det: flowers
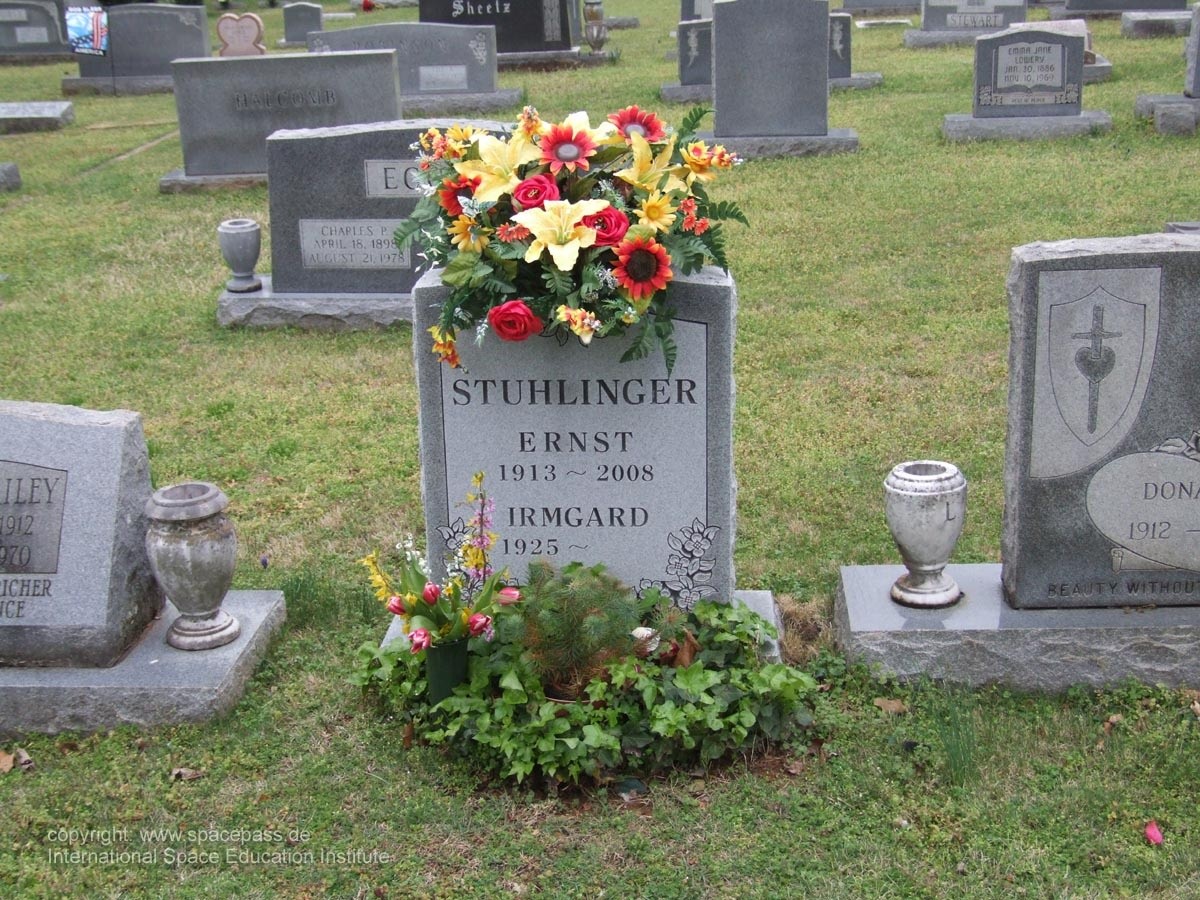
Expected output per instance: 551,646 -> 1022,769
390,104 -> 750,379
356,470 -> 521,654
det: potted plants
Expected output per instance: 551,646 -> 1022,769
498,560 -> 688,718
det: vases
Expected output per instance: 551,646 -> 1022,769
424,631 -> 472,743
